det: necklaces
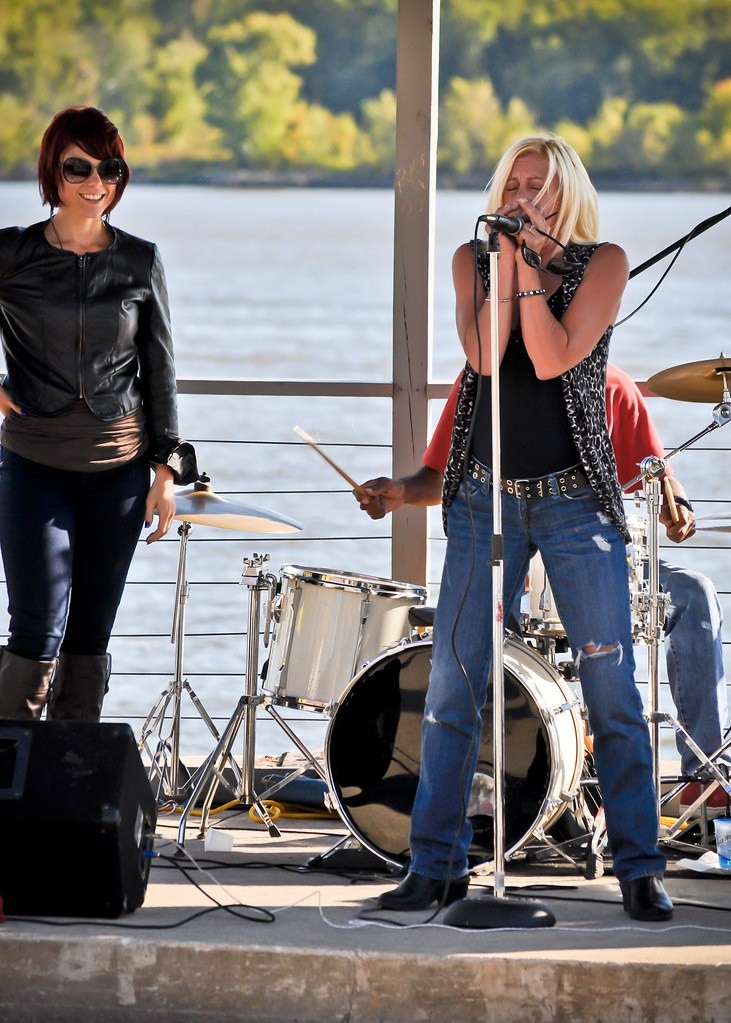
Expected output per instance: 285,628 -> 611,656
50,215 -> 65,250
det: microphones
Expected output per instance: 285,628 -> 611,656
479,214 -> 526,237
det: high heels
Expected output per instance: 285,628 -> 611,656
378,872 -> 471,911
621,877 -> 675,921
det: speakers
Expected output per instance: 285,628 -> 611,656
0,718 -> 159,919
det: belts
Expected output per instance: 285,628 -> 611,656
466,456 -> 589,501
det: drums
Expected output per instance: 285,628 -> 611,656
263,562 -> 429,714
323,633 -> 584,875
529,514 -> 647,632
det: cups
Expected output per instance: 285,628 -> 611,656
713,817 -> 731,870
203,827 -> 233,852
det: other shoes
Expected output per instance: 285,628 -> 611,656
678,781 -> 730,815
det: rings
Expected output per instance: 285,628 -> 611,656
528,225 -> 535,233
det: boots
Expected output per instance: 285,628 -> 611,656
46,650 -> 111,721
0,645 -> 59,719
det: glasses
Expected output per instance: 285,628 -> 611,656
521,212 -> 584,277
57,157 -> 125,185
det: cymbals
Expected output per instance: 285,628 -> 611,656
645,358 -> 731,403
152,490 -> 305,533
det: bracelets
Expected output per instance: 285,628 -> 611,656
515,286 -> 545,297
483,298 -> 511,303
666,495 -> 693,512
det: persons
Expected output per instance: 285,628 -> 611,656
353,362 -> 731,824
378,135 -> 678,920
0,107 -> 199,720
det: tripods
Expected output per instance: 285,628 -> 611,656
584,370 -> 731,880
135,520 -> 332,856
